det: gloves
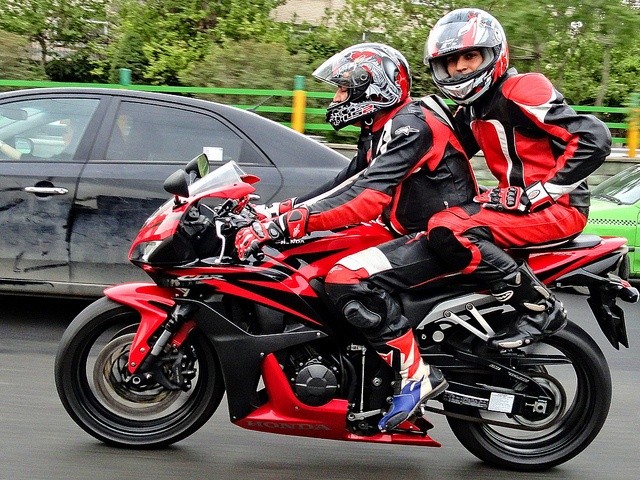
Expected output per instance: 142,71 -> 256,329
234,211 -> 291,261
248,201 -> 280,219
473,186 -> 530,214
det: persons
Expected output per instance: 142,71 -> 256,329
234,42 -> 479,433
425,7 -> 612,353
0,118 -> 86,161
114,115 -> 166,163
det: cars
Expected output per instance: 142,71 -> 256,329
0,85 -> 354,305
579,164 -> 640,277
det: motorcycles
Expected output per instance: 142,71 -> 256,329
53,153 -> 639,470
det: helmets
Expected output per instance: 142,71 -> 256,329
310,42 -> 413,133
422,7 -> 510,107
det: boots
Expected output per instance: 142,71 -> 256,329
369,328 -> 449,433
485,256 -> 569,350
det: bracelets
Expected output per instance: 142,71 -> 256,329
0,140 -> 5,149
